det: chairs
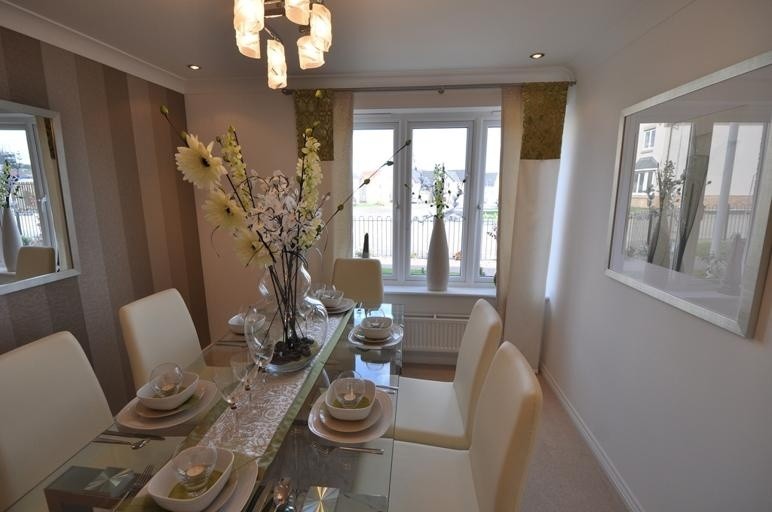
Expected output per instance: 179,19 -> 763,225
365,299 -> 502,449
118,288 -> 244,424
3,293 -> 405,512
0,330 -> 188,511
331,259 -> 383,309
16,246 -> 56,280
326,341 -> 542,511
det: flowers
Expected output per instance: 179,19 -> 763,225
403,163 -> 468,220
0,160 -> 24,207
158,103 -> 413,354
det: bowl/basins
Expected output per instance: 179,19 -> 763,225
334,369 -> 366,408
227,312 -> 266,335
150,363 -> 183,397
325,378 -> 377,421
172,436 -> 217,497
360,317 -> 393,339
316,290 -> 344,307
365,308 -> 385,327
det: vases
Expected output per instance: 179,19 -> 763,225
1,205 -> 24,271
244,252 -> 325,375
427,216 -> 450,292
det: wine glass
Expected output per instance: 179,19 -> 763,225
229,350 -> 261,415
249,336 -> 275,395
213,370 -> 247,435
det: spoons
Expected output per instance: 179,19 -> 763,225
272,485 -> 289,512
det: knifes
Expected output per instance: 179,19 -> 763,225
102,430 -> 166,440
250,478 -> 274,512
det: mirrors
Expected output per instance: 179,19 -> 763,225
604,53 -> 772,340
0,100 -> 82,297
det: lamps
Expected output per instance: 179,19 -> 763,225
232,0 -> 334,90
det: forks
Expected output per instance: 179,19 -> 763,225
112,464 -> 153,511
311,441 -> 384,456
93,436 -> 149,450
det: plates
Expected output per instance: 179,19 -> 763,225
307,388 -> 394,443
202,466 -> 241,511
130,451 -> 259,512
319,397 -> 383,432
136,372 -> 200,411
147,446 -> 234,512
135,383 -> 206,418
328,298 -> 353,314
347,325 -> 403,349
354,326 -> 391,343
116,379 -> 217,431
326,299 -> 349,311
